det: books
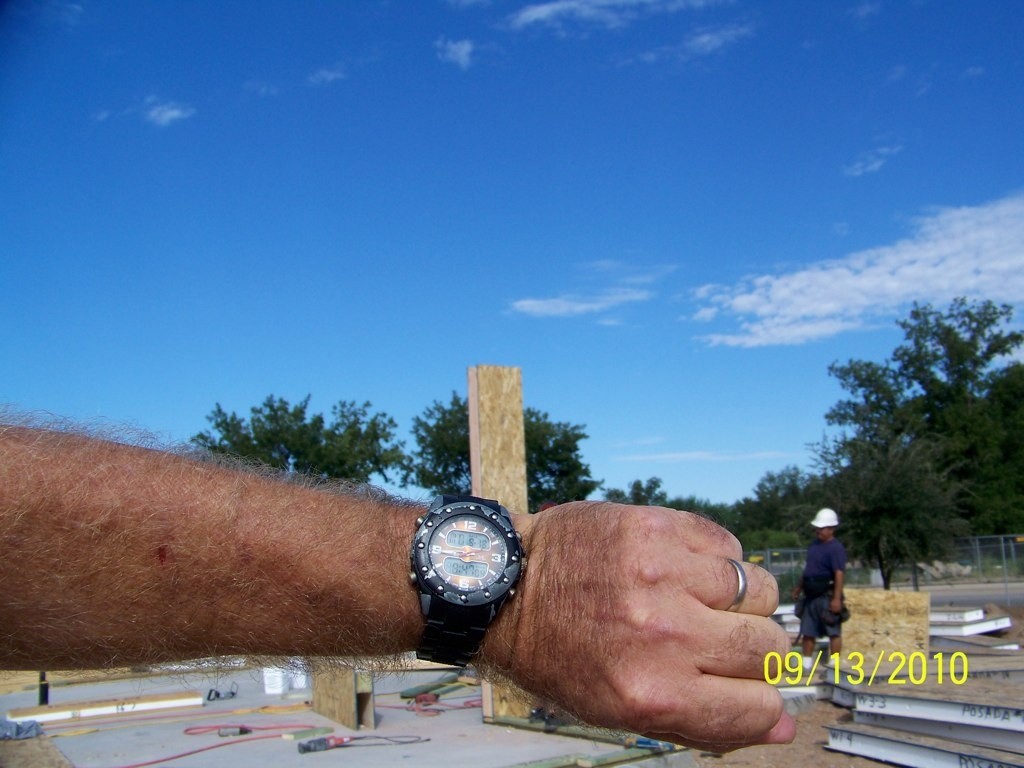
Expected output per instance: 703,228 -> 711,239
770,599 -> 1024,767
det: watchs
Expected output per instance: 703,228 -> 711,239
407,489 -> 530,672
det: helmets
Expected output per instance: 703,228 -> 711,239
810,507 -> 839,528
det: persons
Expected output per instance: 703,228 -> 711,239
791,506 -> 852,671
1,419 -> 800,757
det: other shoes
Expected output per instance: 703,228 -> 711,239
824,656 -> 838,667
801,657 -> 814,670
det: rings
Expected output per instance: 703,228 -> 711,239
724,557 -> 748,612
